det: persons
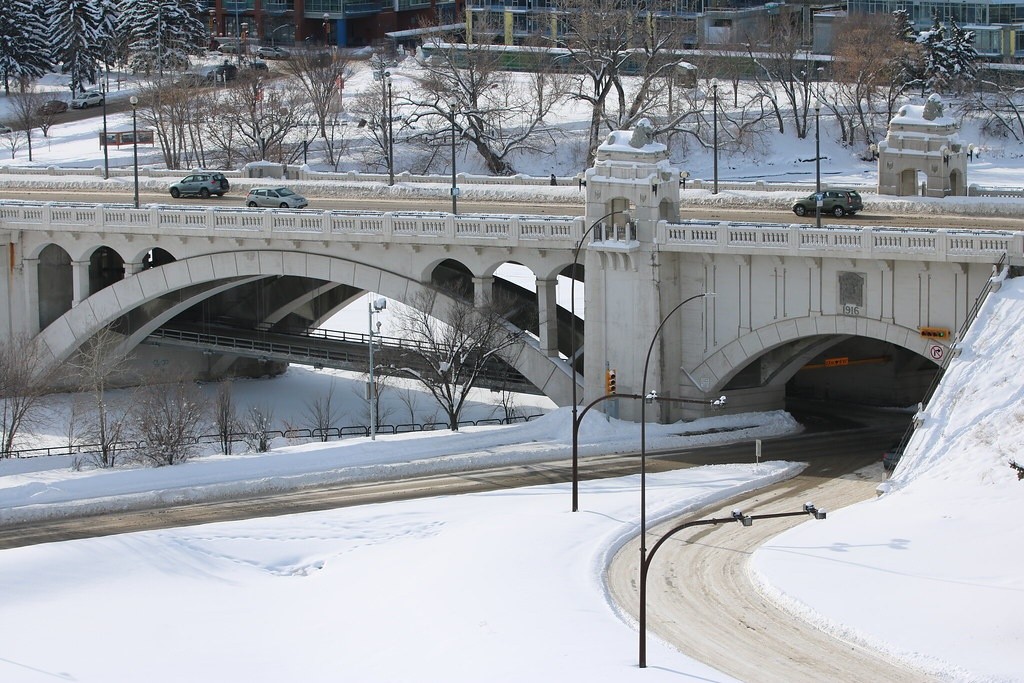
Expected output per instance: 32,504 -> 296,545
551,174 -> 557,186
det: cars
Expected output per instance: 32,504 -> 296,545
37,100 -> 67,114
217,42 -> 245,53
257,47 -> 290,60
0,124 -> 13,133
208,65 -> 238,81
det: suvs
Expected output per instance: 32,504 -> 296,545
72,92 -> 105,109
241,63 -> 268,80
792,188 -> 864,217
169,172 -> 229,199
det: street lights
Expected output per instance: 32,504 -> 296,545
711,77 -> 719,194
448,97 -> 459,216
302,136 -> 307,164
640,291 -> 719,668
815,101 -> 821,229
272,24 -> 289,47
323,13 -> 330,51
570,210 -> 631,512
129,96 -> 140,209
386,76 -> 395,186
260,131 -> 267,160
100,77 -> 109,179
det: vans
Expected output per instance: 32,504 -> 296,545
245,188 -> 308,209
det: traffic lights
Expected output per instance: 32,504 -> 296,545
604,368 -> 617,396
920,326 -> 950,339
